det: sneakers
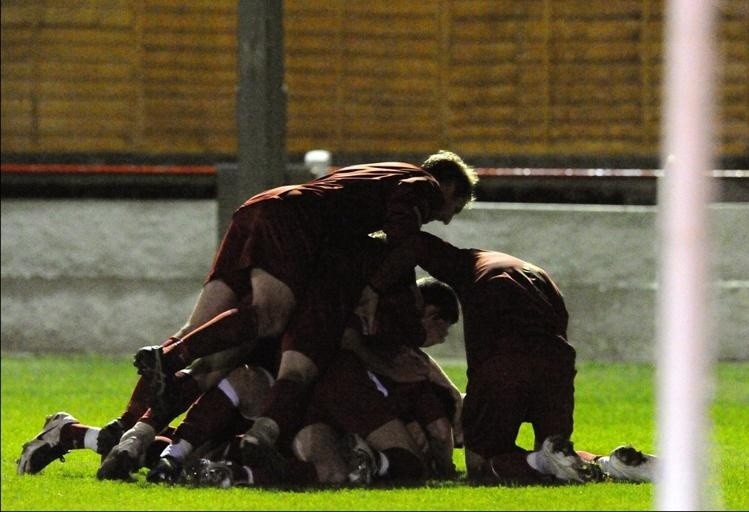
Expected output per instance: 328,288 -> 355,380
18,413 -> 80,473
541,437 -> 655,482
133,345 -> 176,413
96,420 -> 260,486
344,430 -> 381,486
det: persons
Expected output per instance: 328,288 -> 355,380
410,231 -> 659,489
16,149 -> 478,492
